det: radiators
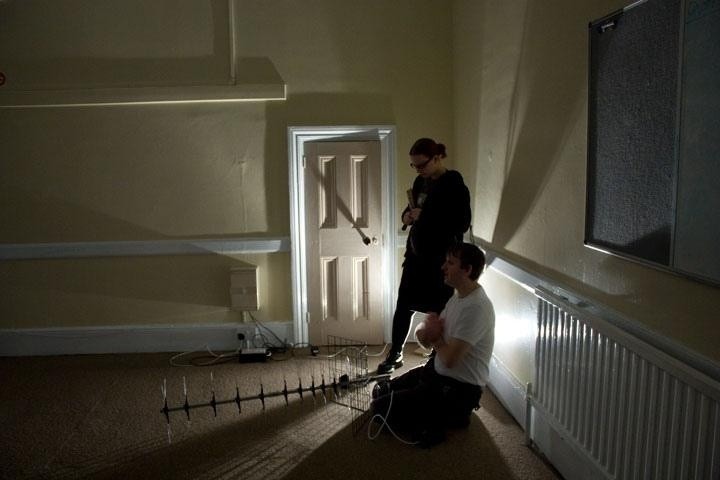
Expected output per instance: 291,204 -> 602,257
524,285 -> 720,480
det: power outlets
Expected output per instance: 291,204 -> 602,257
236,326 -> 256,340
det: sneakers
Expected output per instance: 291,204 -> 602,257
378,350 -> 403,373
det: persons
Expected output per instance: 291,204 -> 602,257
377,138 -> 471,374
375,243 -> 495,430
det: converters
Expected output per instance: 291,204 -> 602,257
239,348 -> 268,363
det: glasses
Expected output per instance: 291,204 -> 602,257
410,157 -> 432,169
